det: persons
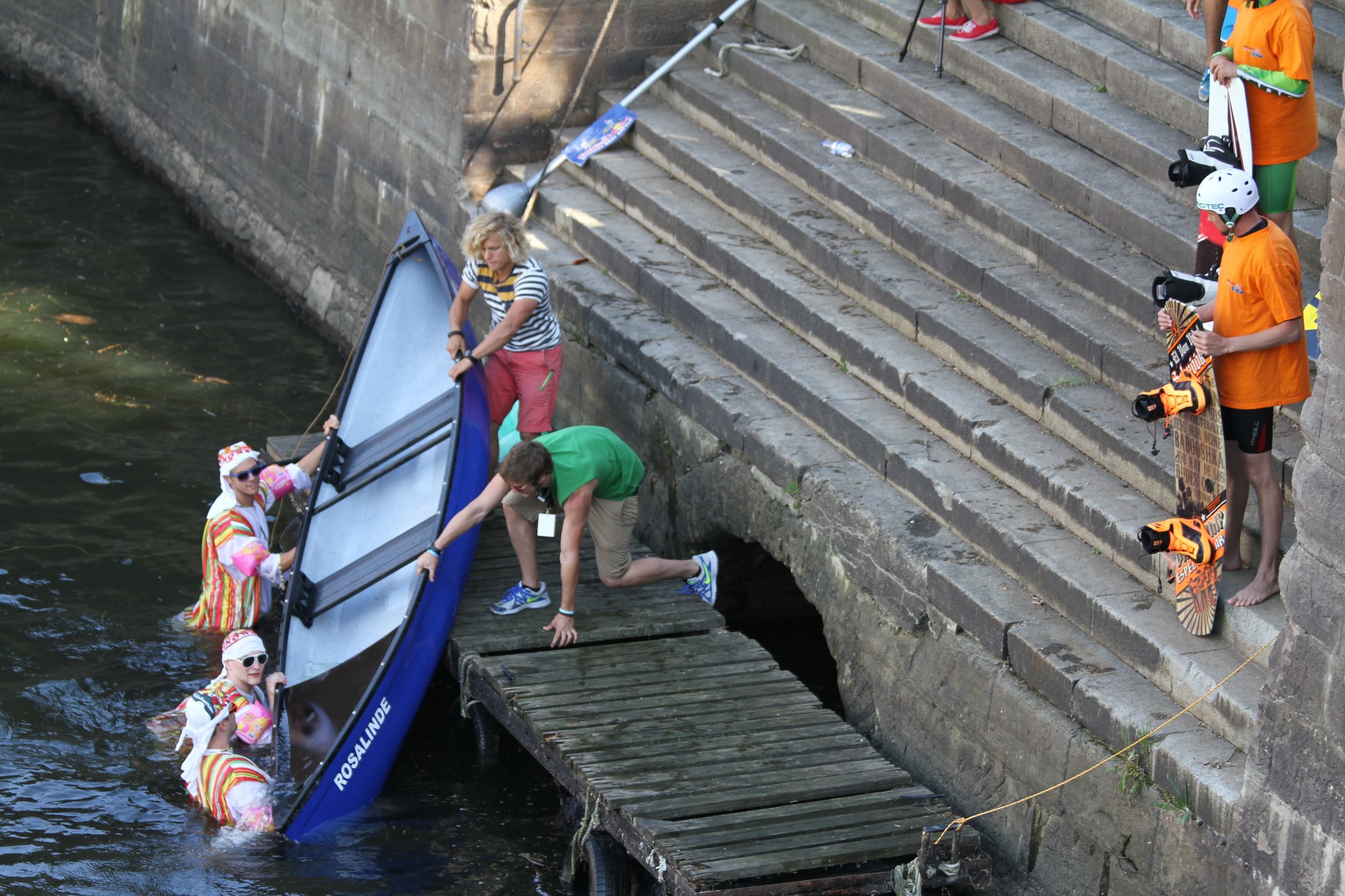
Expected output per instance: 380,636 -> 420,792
1157,168 -> 1313,608
175,414 -> 343,631
415,426 -> 720,651
1182,0 -> 1315,102
915,0 -> 1030,44
146,629 -> 288,748
1205,0 -> 1321,247
447,211 -> 564,442
175,690 -> 278,841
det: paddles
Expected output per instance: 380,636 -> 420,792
521,0 -> 618,227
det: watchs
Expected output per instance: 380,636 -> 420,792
426,542 -> 443,559
461,349 -> 478,364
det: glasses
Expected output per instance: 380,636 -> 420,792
234,652 -> 268,668
227,700 -> 238,716
230,461 -> 262,481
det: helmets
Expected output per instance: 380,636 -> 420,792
1195,168 -> 1260,216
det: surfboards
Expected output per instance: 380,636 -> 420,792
1151,64 -> 1254,332
1131,298 -> 1227,637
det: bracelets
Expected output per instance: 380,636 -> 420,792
557,605 -> 576,618
446,330 -> 464,338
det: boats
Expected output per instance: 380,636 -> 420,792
270,207 -> 491,843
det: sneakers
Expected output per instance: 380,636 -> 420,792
678,550 -> 719,607
1198,67 -> 1210,101
1137,518 -> 1213,593
1132,381 -> 1209,456
948,16 -> 1000,42
490,579 -> 551,616
1167,136 -> 1241,189
917,9 -> 970,30
1152,270 -> 1218,307
